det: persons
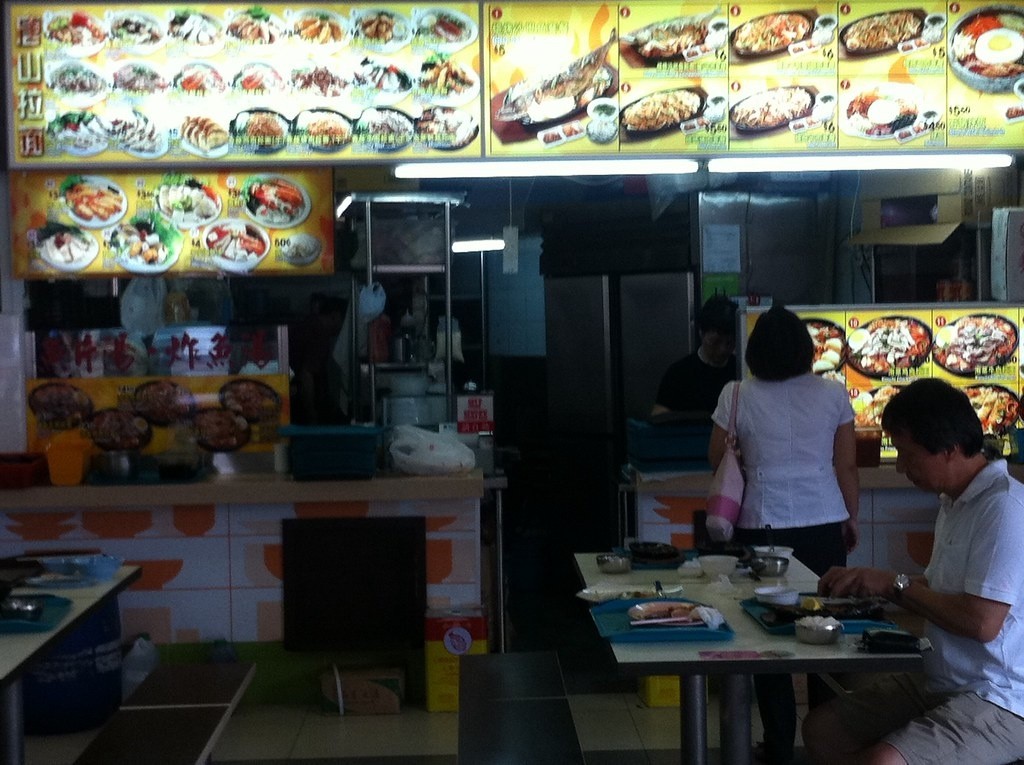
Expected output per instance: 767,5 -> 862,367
708,304 -> 861,764
650,291 -> 739,417
818,376 -> 1024,765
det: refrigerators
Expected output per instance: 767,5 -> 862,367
543,270 -> 692,423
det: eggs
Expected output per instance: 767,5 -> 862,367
974,28 -> 1024,65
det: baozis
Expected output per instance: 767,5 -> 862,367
867,99 -> 899,125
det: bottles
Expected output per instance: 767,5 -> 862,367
398,310 -> 416,360
367,314 -> 392,355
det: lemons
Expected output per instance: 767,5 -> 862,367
801,597 -> 820,611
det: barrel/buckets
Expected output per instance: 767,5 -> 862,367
20,592 -> 122,736
43,438 -> 91,486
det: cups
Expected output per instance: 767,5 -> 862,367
813,91 -> 836,121
705,18 -> 729,50
853,427 -> 882,468
922,13 -> 946,42
1013,78 -> 1024,100
705,93 -> 726,123
584,97 -> 619,142
811,14 -> 838,44
917,108 -> 942,123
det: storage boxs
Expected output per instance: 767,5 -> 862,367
749,673 -> 807,705
319,666 -> 405,717
632,666 -> 709,710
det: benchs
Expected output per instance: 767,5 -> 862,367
71,661 -> 257,765
458,650 -> 586,765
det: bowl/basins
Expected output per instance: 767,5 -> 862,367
596,541 -> 678,574
203,219 -> 270,273
797,314 -> 847,373
699,555 -> 739,581
966,382 -> 1019,433
751,556 -> 789,578
933,312 -> 1019,376
845,315 -> 932,378
43,555 -> 123,582
47,108 -> 480,158
754,586 -> 799,604
41,4 -> 478,55
26,378 -> 286,453
794,621 -> 841,646
276,426 -> 385,482
947,4 -> 1024,94
0,598 -> 45,622
41,58 -> 481,109
280,232 -> 322,263
755,545 -> 794,557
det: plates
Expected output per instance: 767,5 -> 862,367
787,39 -> 819,56
1002,105 -> 1024,124
619,85 -> 709,137
537,119 -> 586,149
682,43 -> 712,62
627,602 -> 707,627
897,38 -> 931,55
237,173 -> 311,227
786,117 -> 820,134
680,116 -> 711,136
838,6 -> 931,55
108,179 -> 223,274
24,575 -> 98,589
835,82 -> 930,139
576,584 -> 684,601
894,125 -> 929,143
729,85 -> 820,134
729,8 -> 821,57
501,62 -> 612,128
631,24 -> 708,60
37,174 -> 128,271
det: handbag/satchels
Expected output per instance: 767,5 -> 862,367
705,380 -> 744,542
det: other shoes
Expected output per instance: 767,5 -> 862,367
754,741 -> 764,765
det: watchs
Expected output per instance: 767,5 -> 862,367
892,572 -> 913,594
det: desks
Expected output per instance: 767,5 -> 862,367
0,565 -> 143,765
569,552 -> 924,765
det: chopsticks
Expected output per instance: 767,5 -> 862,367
629,615 -> 689,626
